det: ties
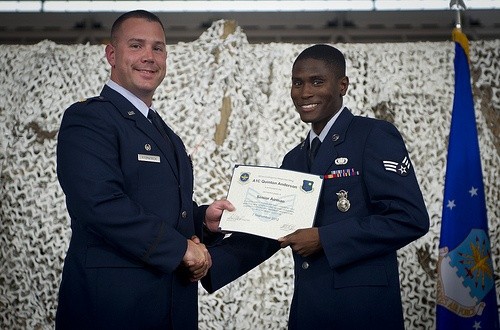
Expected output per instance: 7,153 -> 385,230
310,136 -> 320,168
148,109 -> 175,155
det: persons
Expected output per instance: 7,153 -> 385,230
188,44 -> 431,330
55,8 -> 236,330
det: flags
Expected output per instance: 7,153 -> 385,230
435,30 -> 500,329
